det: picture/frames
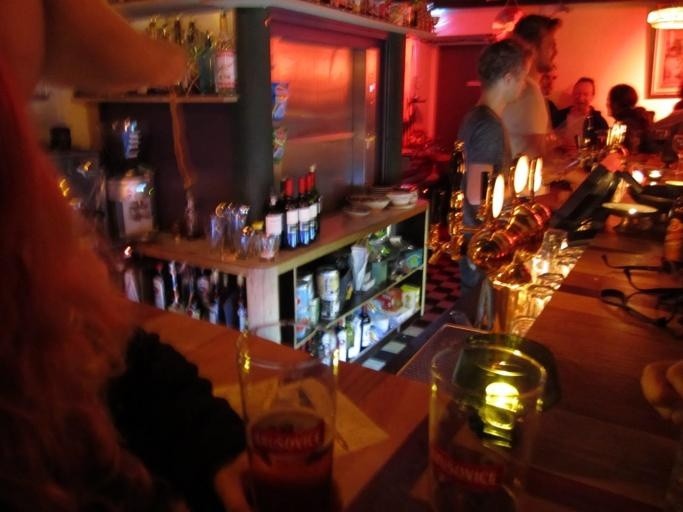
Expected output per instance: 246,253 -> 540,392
642,3 -> 683,100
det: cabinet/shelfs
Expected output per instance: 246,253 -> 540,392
127,194 -> 432,372
67,1 -> 238,241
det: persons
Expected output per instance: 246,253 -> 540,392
557,78 -> 655,154
459,13 -> 558,294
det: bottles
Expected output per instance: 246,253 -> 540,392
660,195 -> 683,268
120,11 -> 322,329
319,302 -> 375,364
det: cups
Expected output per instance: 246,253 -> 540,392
430,227 -> 586,503
234,322 -> 337,504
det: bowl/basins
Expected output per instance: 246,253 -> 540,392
342,191 -> 415,218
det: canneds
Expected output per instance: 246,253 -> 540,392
295,263 -> 340,341
